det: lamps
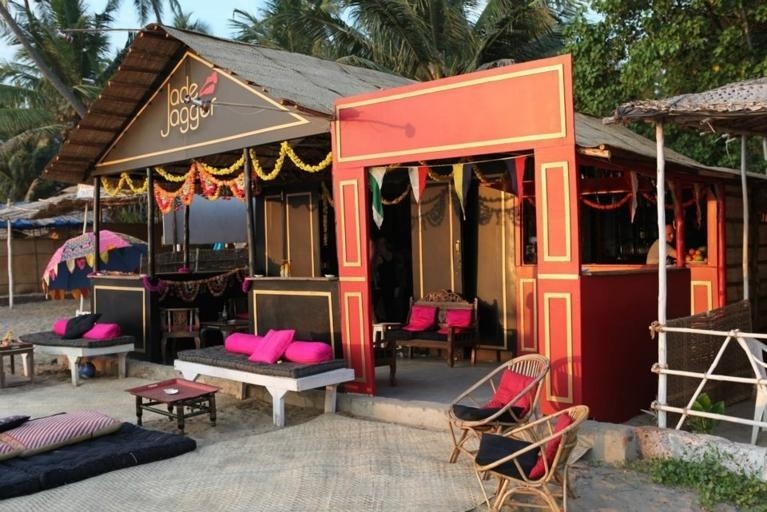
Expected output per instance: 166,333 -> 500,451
191,98 -> 314,118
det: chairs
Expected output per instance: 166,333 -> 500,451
443,353 -> 549,461
475,404 -> 591,511
159,308 -> 202,357
371,329 -> 399,385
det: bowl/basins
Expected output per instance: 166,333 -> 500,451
233,242 -> 246,250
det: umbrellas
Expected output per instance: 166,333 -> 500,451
42,230 -> 148,304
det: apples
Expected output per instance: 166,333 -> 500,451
685,249 -> 703,261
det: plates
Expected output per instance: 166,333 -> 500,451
684,260 -> 707,265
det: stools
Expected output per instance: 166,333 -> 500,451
371,322 -> 403,356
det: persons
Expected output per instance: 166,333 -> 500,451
646,223 -> 676,265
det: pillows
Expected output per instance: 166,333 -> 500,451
0,410 -> 122,455
437,309 -> 472,335
224,331 -> 331,364
53,320 -> 123,340
528,413 -> 573,480
401,305 -> 436,333
63,312 -> 101,340
0,414 -> 30,437
453,403 -> 522,423
249,329 -> 295,363
485,368 -> 536,416
473,433 -> 538,479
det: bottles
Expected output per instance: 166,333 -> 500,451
175,242 -> 183,266
213,240 -> 228,250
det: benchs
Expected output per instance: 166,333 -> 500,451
388,288 -> 479,367
17,331 -> 136,387
171,344 -> 355,428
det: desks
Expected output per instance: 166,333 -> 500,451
127,375 -> 221,433
202,321 -> 249,344
0,345 -> 36,389
